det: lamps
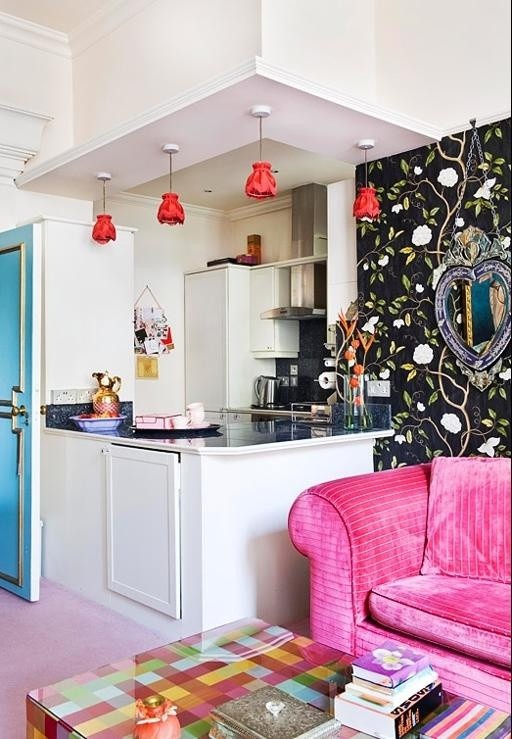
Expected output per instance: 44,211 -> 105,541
244,103 -> 278,202
91,171 -> 118,246
352,138 -> 382,225
156,143 -> 187,228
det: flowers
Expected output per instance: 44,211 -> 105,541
333,305 -> 377,430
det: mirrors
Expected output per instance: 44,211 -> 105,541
427,226 -> 512,372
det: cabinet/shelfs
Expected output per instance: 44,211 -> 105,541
248,266 -> 301,360
101,445 -> 182,621
184,262 -> 277,412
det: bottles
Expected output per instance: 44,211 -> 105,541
133,696 -> 180,738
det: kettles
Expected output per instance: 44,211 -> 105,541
254,375 -> 282,405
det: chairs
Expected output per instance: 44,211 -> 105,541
286,462 -> 512,716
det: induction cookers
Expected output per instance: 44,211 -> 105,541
250,406 -> 315,412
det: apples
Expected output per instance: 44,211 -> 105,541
101,411 -> 119,418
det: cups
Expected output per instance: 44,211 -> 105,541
171,415 -> 192,429
186,402 -> 206,424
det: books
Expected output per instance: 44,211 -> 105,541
208,685 -> 341,739
418,697 -> 511,739
334,639 -> 442,739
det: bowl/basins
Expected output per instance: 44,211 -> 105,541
69,415 -> 127,430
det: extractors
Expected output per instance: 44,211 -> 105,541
259,182 -> 328,321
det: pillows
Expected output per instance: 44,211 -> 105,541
420,455 -> 512,587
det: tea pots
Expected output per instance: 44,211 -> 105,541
90,370 -> 123,415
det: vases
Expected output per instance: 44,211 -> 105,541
341,370 -> 371,431
